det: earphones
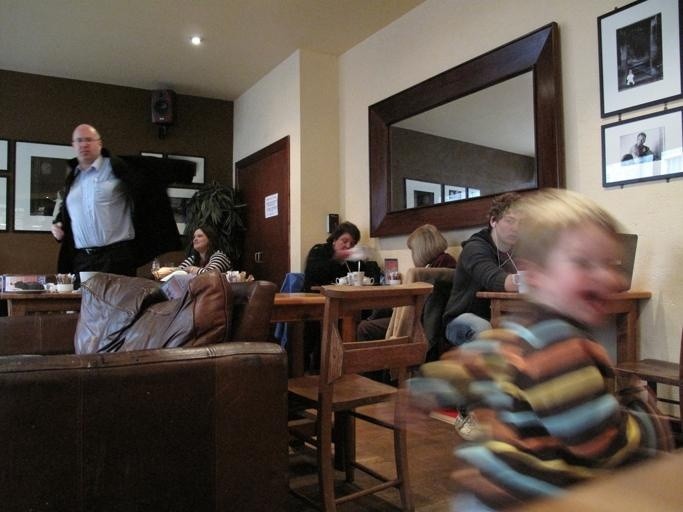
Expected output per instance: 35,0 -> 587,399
493,220 -> 496,224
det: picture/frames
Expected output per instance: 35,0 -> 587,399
1,139 -> 205,237
403,177 -> 482,208
597,0 -> 683,187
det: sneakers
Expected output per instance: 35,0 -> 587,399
454,414 -> 482,441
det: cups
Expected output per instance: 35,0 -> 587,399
42,283 -> 74,294
388,272 -> 401,285
335,272 -> 374,286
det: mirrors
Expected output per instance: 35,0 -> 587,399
369,21 -> 565,240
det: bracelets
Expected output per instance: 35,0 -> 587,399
190,267 -> 195,273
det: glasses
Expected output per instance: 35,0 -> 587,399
72,137 -> 98,143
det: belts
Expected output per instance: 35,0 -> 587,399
80,247 -> 101,255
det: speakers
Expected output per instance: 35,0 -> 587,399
148,88 -> 177,126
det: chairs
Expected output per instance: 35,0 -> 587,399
289,283 -> 434,512
607,323 -> 682,449
1,279 -> 288,512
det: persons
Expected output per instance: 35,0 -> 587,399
420,194 -> 432,208
174,224 -> 234,280
455,190 -> 461,201
623,132 -> 659,163
442,192 -> 531,348
356,224 -> 456,385
301,220 -> 373,375
627,68 -> 638,87
50,124 -> 197,289
405,188 -> 678,511
449,191 -> 455,202
